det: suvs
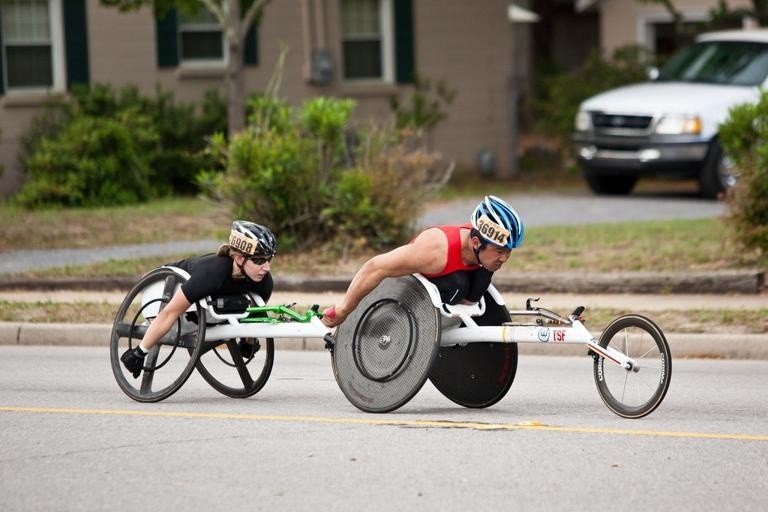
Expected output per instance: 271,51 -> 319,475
572,28 -> 768,198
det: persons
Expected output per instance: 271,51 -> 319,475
119,219 -> 278,378
315,194 -> 525,330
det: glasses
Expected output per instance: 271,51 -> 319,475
239,253 -> 273,266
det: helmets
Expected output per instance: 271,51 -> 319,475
227,219 -> 278,260
469,193 -> 524,251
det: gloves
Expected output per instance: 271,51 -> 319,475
119,344 -> 148,380
235,337 -> 261,360
